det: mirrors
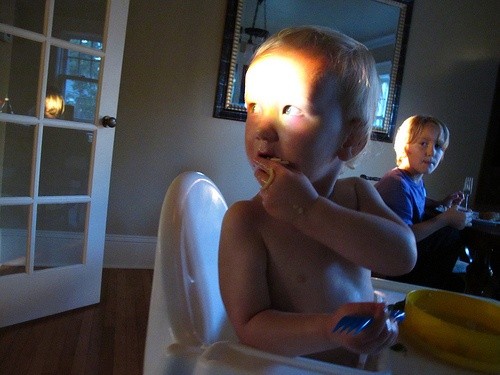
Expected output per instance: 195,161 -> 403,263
212,0 -> 415,144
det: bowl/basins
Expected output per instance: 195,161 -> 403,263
404,288 -> 500,375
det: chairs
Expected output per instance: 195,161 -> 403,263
143,171 -> 374,375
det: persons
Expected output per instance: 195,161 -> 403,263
371,114 -> 473,282
219,25 -> 418,372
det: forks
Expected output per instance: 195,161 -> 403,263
331,297 -> 405,337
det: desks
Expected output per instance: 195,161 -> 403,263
419,199 -> 500,235
351,276 -> 500,375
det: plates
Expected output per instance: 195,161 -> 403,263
435,206 -> 473,212
472,211 -> 500,224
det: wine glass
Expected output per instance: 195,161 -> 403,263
459,176 -> 473,211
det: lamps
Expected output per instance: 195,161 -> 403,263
244,0 -> 270,65
